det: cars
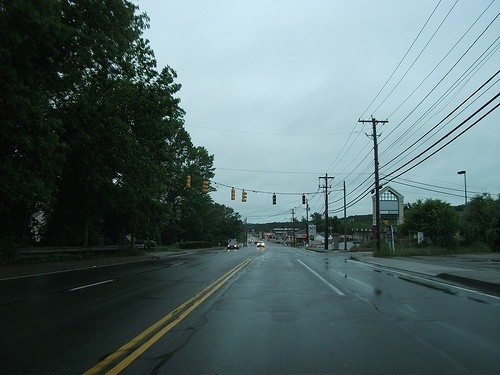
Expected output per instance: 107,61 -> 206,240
248,239 -> 259,244
133,241 -> 149,250
228,241 -> 240,249
257,241 -> 265,247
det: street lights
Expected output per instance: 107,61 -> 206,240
457,171 -> 468,204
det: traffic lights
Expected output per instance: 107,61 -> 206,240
273,194 -> 277,205
241,191 -> 247,202
202,181 -> 209,193
302,195 -> 306,205
